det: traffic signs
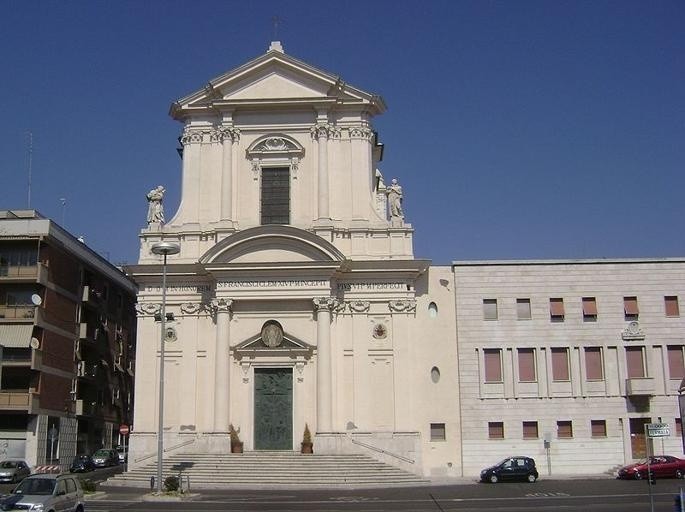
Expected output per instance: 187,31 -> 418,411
648,424 -> 671,437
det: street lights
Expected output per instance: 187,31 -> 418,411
152,241 -> 181,493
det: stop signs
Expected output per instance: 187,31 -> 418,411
120,425 -> 129,435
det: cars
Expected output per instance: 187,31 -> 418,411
480,456 -> 538,483
1,473 -> 86,512
0,461 -> 31,484
618,455 -> 684,480
70,446 -> 129,473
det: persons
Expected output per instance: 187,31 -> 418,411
145,186 -> 166,224
385,176 -> 407,220
